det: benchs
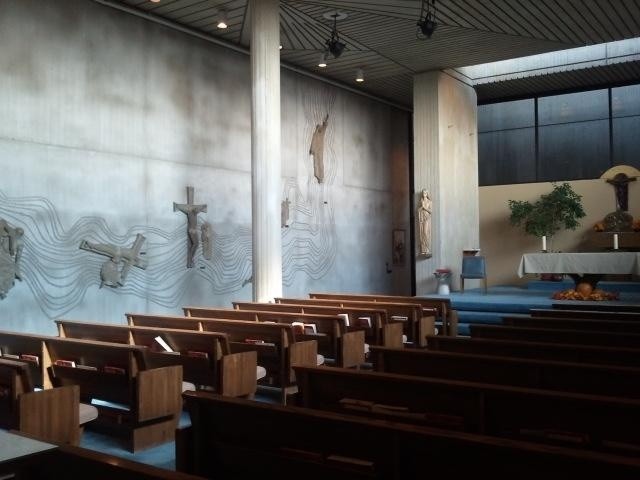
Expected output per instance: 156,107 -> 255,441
0,289 -> 640,480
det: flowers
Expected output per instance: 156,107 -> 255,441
432,268 -> 451,281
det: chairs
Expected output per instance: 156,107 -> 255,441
459,255 -> 488,295
593,210 -> 640,252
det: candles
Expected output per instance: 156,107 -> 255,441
613,233 -> 619,249
541,235 -> 547,250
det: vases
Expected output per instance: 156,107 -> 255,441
436,280 -> 449,295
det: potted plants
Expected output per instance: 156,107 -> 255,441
506,181 -> 587,280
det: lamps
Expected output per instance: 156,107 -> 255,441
215,9 -> 228,30
355,67 -> 364,82
319,52 -> 327,69
416,0 -> 440,41
324,13 -> 345,58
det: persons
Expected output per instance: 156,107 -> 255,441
605,174 -> 635,211
416,189 -> 433,255
0,202 -> 212,303
307,119 -> 329,182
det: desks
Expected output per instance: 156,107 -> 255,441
521,252 -> 640,293
